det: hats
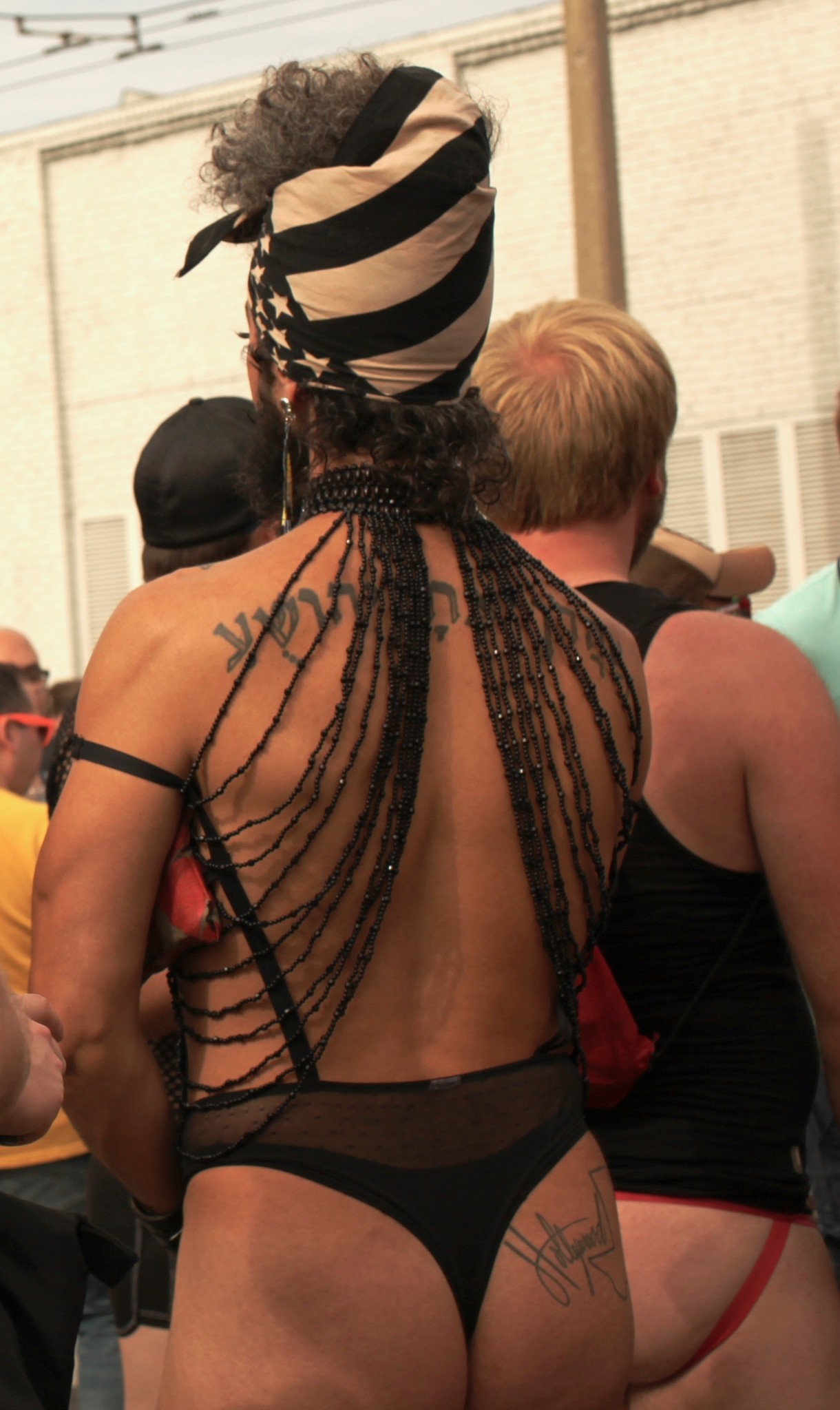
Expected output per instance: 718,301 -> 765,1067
627,523 -> 776,610
134,397 -> 285,552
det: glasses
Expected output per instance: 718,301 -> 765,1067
0,710 -> 65,748
13,664 -> 48,691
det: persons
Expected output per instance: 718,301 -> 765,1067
1,57 -> 838,1409
